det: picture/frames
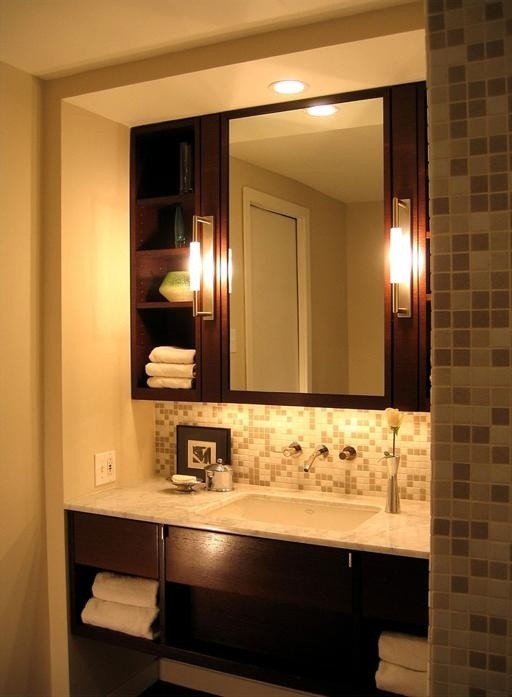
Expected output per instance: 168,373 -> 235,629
175,424 -> 231,484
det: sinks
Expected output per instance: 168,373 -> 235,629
190,483 -> 385,537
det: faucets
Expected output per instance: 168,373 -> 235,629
303,447 -> 329,473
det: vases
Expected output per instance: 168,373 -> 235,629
384,455 -> 402,514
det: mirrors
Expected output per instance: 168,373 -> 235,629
222,84 -> 396,410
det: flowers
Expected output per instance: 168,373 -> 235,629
383,405 -> 405,459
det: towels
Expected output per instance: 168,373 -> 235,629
144,345 -> 197,390
374,630 -> 430,697
80,570 -> 161,642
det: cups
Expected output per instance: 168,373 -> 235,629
176,141 -> 194,194
204,459 -> 235,491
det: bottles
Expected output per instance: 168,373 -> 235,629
173,205 -> 187,247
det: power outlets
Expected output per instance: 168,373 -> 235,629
94,451 -> 118,487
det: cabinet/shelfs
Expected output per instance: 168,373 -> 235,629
129,115 -> 204,403
63,508 -> 430,697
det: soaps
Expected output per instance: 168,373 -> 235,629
170,473 -> 198,485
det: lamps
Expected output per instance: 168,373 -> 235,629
189,214 -> 216,321
389,196 -> 413,319
